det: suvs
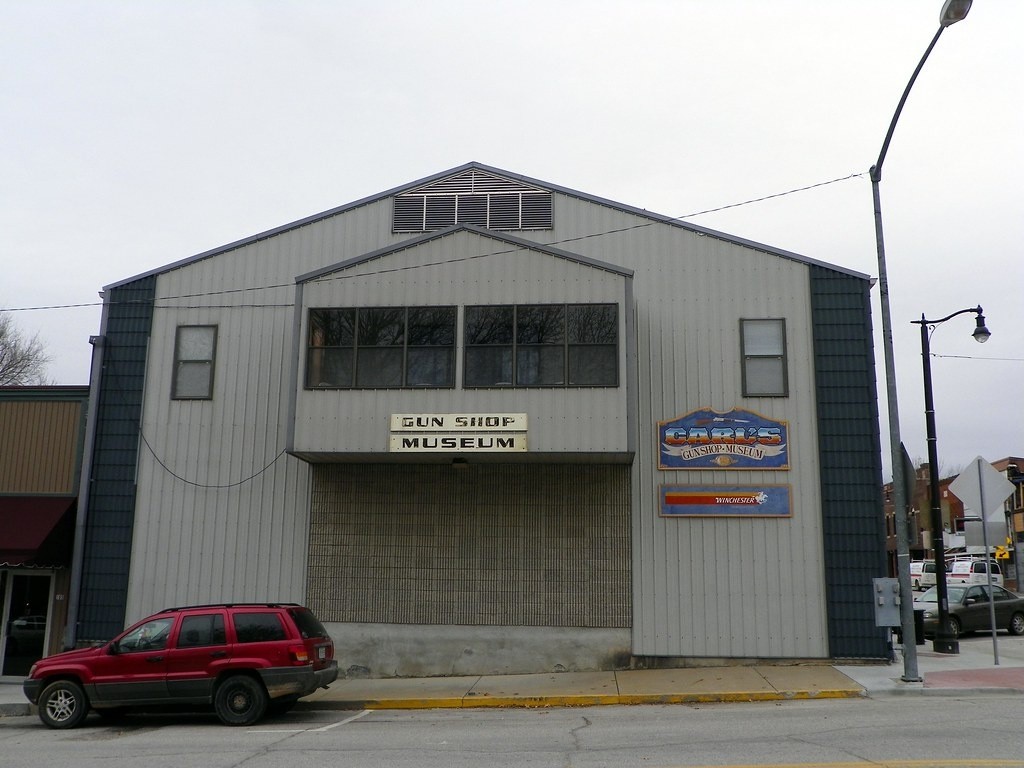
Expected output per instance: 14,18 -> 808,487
23,603 -> 339,729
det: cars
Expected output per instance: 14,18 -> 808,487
913,581 -> 1024,638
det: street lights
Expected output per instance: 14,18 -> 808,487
911,306 -> 993,656
867,1 -> 975,684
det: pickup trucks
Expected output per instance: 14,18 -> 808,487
908,557 -> 1005,590
